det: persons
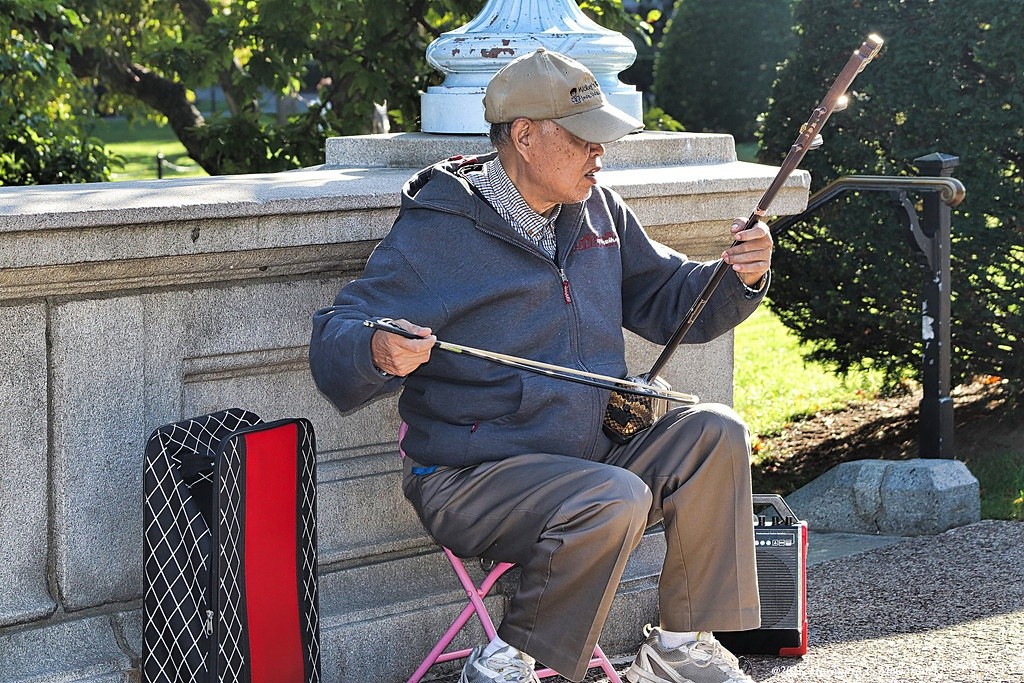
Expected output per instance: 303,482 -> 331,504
309,50 -> 773,683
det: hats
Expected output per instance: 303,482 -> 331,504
483,47 -> 645,143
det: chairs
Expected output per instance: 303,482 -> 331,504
394,421 -> 626,683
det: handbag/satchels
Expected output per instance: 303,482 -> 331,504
142,408 -> 321,683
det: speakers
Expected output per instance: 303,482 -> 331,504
711,515 -> 808,658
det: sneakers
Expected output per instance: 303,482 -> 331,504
457,645 -> 541,683
625,623 -> 756,683
